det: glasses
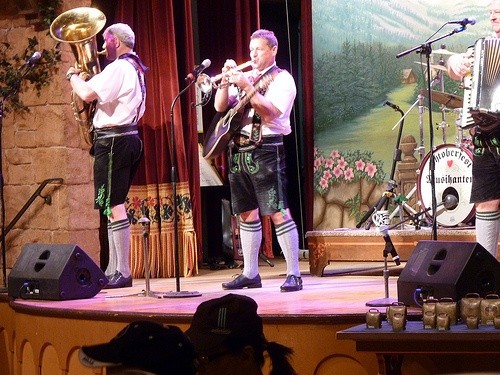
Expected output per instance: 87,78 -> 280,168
194,349 -> 240,365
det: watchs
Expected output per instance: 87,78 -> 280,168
67,72 -> 76,81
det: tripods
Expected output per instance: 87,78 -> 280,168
357,100 -> 418,230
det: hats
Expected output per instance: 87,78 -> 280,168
77,319 -> 195,375
184,293 -> 264,351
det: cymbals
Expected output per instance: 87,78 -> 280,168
429,48 -> 456,56
414,62 -> 449,73
419,88 -> 464,109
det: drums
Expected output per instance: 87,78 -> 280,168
416,142 -> 477,228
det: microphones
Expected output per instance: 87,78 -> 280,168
443,194 -> 458,210
185,59 -> 211,82
379,224 -> 401,266
18,52 -> 41,71
448,18 -> 476,25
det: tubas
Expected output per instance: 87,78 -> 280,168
50,7 -> 107,145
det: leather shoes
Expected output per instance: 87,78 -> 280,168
103,272 -> 132,289
280,275 -> 304,293
222,274 -> 263,289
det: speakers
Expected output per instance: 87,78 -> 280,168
397,239 -> 500,309
8,244 -> 110,301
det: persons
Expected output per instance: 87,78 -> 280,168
445,0 -> 500,262
214,31 -> 305,292
79,293 -> 296,375
66,23 -> 145,289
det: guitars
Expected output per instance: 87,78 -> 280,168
200,75 -> 273,160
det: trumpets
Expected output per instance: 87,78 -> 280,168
196,59 -> 255,94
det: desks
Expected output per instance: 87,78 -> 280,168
336,321 -> 500,375
